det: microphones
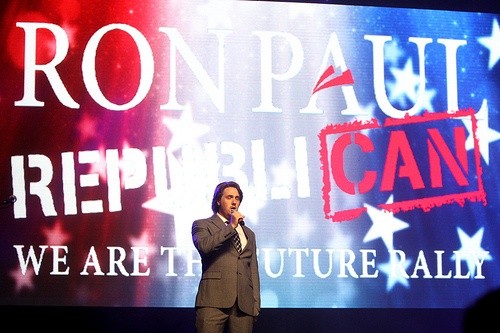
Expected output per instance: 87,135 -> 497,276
231,210 -> 244,225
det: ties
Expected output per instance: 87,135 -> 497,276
226,221 -> 242,254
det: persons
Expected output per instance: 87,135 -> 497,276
191,180 -> 262,333
